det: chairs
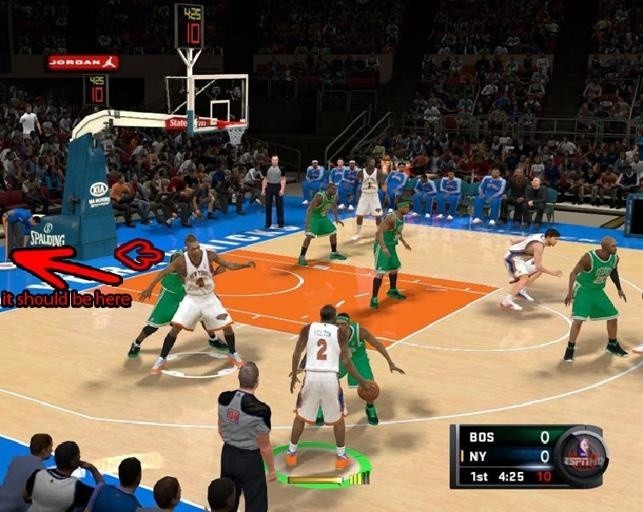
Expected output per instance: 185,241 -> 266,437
0,126 -> 195,225
302,170 -> 557,229
256,64 -> 643,141
